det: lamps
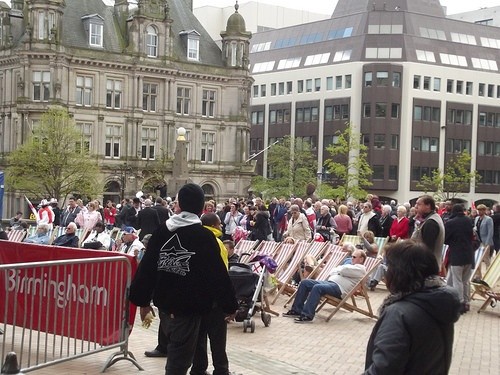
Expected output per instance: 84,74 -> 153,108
441,126 -> 448,129
176,127 -> 187,140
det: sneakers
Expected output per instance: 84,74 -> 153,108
282,310 -> 299,317
294,315 -> 314,324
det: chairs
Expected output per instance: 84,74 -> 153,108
7,225 -> 142,253
234,233 -> 500,324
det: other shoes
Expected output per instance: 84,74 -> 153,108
229,372 -> 243,375
368,279 -> 379,290
144,348 -> 168,357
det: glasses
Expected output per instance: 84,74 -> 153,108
351,256 -> 357,258
88,205 -> 93,207
66,227 -> 71,230
364,206 -> 368,208
415,203 -> 424,206
123,233 -> 126,236
291,212 -> 296,215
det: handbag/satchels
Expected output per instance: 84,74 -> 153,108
85,241 -> 103,249
329,228 -> 339,244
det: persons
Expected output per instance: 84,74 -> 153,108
360,239 -> 462,375
0,192 -> 500,313
128,183 -> 241,375
282,249 -> 367,324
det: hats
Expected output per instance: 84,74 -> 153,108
178,183 -> 205,214
39,199 -> 50,206
122,227 -> 135,234
133,197 -> 141,203
166,196 -> 172,203
49,198 -> 58,203
141,233 -> 152,243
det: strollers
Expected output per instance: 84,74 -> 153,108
227,252 -> 272,334
